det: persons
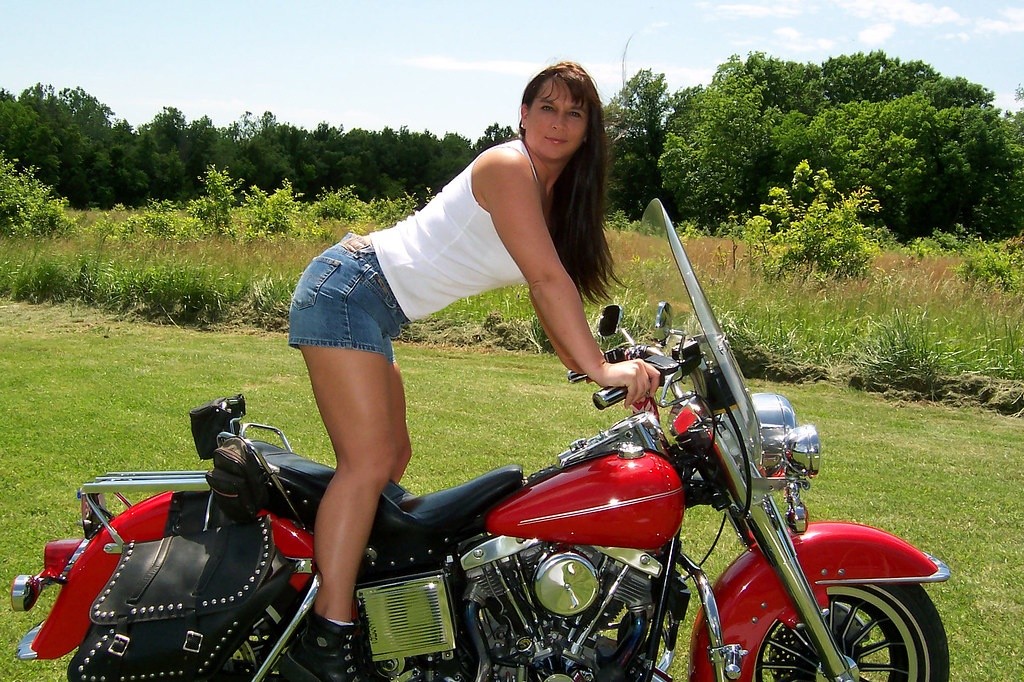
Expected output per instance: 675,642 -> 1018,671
288,63 -> 661,682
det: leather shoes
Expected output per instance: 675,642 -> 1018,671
279,613 -> 369,682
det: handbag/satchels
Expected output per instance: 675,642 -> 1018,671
190,393 -> 246,461
205,435 -> 268,521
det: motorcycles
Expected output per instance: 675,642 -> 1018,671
7,198 -> 955,682
